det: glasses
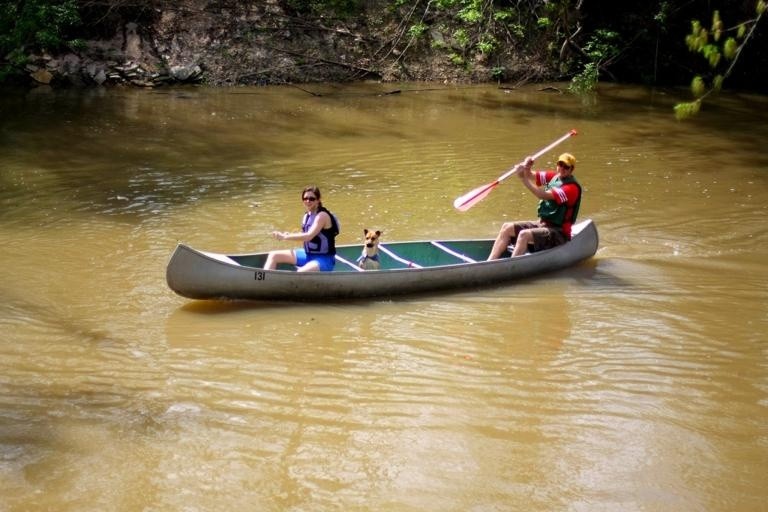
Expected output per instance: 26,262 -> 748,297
303,197 -> 316,201
557,161 -> 570,170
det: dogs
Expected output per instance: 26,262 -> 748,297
358,229 -> 381,270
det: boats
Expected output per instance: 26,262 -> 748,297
166,218 -> 597,299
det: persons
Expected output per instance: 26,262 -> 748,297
486,152 -> 582,261
263,185 -> 340,272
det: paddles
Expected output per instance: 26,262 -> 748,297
454,130 -> 576,212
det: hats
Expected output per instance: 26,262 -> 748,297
557,152 -> 576,168
